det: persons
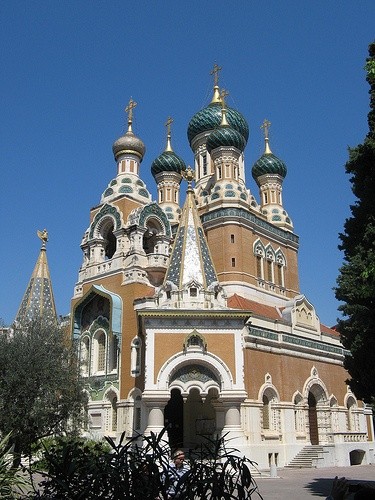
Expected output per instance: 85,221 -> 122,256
157,450 -> 192,500
